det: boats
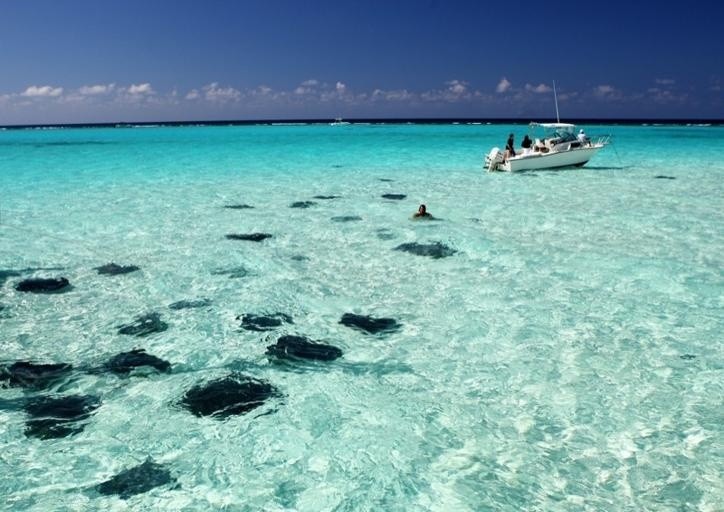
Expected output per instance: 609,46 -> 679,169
484,118 -> 612,176
329,121 -> 349,126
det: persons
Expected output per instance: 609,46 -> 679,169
521,135 -> 532,153
576,128 -> 591,147
506,133 -> 515,157
413,205 -> 431,220
